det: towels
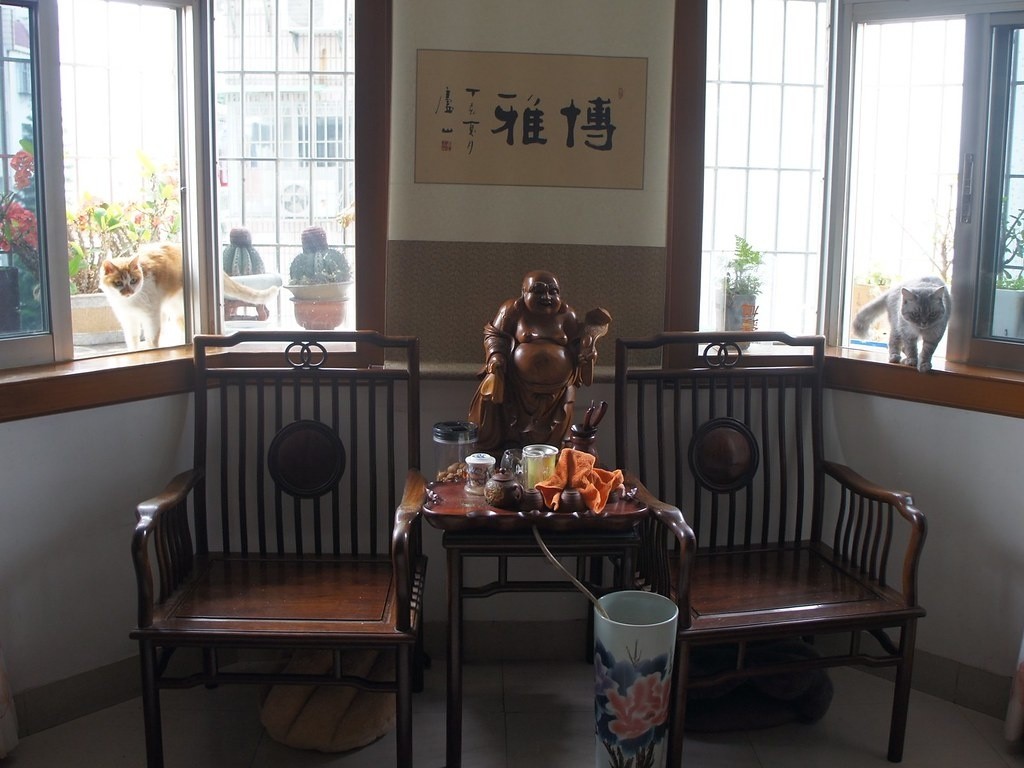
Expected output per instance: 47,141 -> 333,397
535,448 -> 625,514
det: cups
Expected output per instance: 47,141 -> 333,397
560,489 -> 584,513
499,448 -> 522,480
522,488 -> 543,512
521,443 -> 559,489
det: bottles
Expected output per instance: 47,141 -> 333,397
432,421 -> 480,486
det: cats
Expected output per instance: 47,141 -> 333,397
852,276 -> 953,373
99,243 -> 279,351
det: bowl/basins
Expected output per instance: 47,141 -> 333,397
289,297 -> 349,330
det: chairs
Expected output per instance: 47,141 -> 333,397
128,329 -> 430,768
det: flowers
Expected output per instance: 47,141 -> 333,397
0,149 -> 39,255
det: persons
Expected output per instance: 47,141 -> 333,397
485,270 -> 598,451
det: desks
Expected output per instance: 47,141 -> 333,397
442,529 -> 642,768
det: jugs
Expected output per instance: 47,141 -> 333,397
483,468 -> 524,509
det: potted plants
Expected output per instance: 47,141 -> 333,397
991,276 -> 1024,338
724,234 -> 765,351
222,228 -> 282,320
850,269 -> 892,342
283,225 -> 354,331
65,191 -> 125,345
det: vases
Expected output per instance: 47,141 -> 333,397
616,332 -> 928,768
593,591 -> 679,768
0,266 -> 22,333
